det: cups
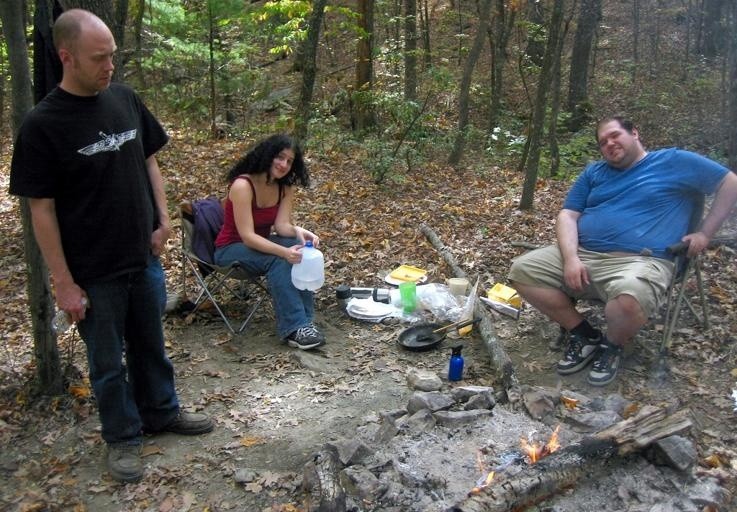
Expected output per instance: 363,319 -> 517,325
400,282 -> 416,314
449,278 -> 469,295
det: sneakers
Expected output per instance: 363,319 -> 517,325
557,329 -> 603,375
287,323 -> 326,350
587,344 -> 623,386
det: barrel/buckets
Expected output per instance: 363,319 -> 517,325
290,241 -> 325,292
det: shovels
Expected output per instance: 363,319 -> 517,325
416,319 -> 469,341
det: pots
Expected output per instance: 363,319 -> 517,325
398,316 -> 482,350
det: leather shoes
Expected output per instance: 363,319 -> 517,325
168,413 -> 213,434
107,430 -> 143,483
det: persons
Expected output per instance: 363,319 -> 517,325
8,7 -> 214,481
507,115 -> 737,386
213,133 -> 326,351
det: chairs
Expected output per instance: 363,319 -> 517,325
180,200 -> 272,335
548,246 -> 708,368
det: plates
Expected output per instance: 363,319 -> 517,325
385,275 -> 428,286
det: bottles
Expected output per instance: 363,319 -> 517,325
49,298 -> 87,333
350,287 -> 389,301
